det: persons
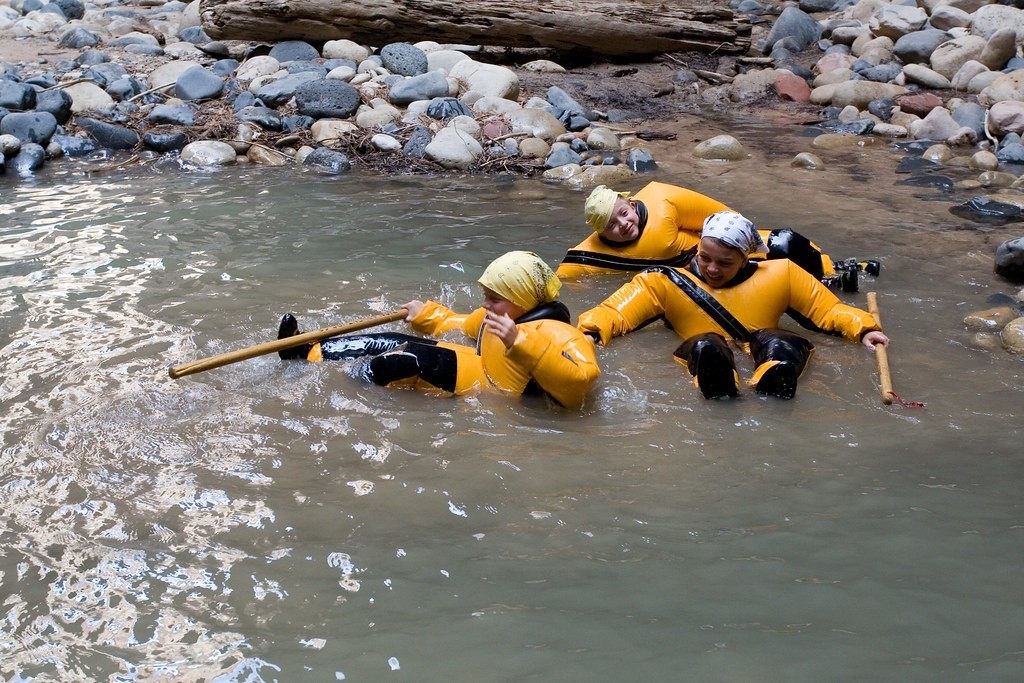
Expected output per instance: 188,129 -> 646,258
576,211 -> 891,400
277,250 -> 601,414
557,181 -> 880,295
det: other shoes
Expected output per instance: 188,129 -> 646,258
696,344 -> 738,398
277,313 -> 310,359
836,258 -> 879,276
823,273 -> 857,294
755,360 -> 796,401
